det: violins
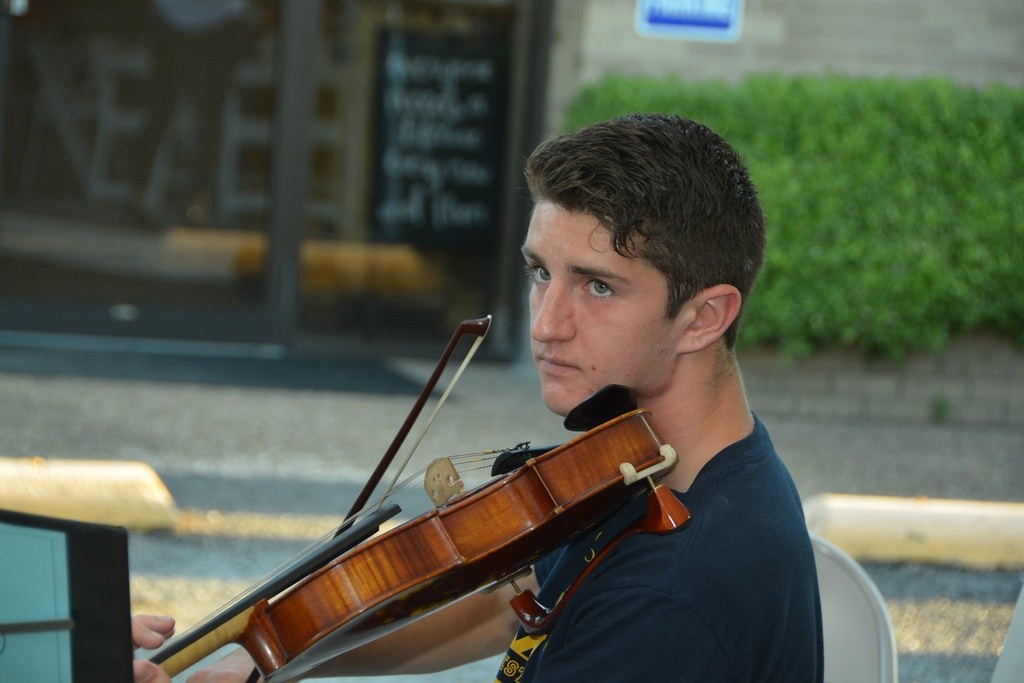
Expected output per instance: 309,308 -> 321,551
110,402 -> 681,683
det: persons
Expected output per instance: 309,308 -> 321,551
130,112 -> 821,683
